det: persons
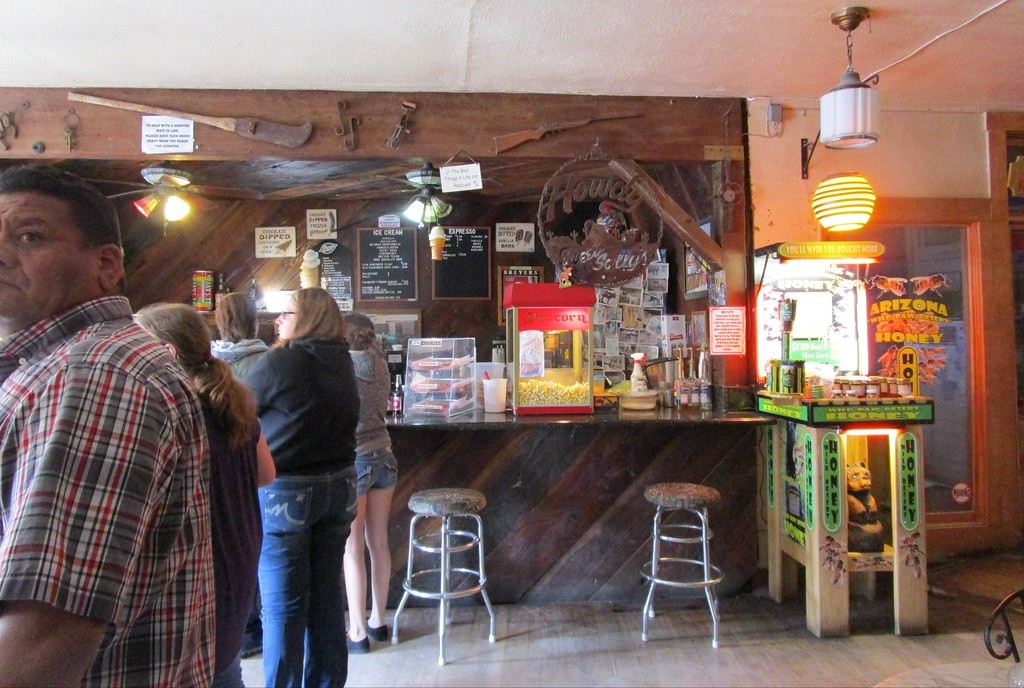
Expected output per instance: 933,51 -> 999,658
209,294 -> 268,657
130,302 -> 266,688
241,289 -> 359,688
1,165 -> 216,688
338,312 -> 399,654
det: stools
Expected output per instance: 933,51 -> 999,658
391,488 -> 495,666
638,483 -> 725,649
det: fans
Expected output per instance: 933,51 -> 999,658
84,168 -> 265,212
376,162 -> 464,196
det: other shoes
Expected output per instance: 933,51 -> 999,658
344,631 -> 369,654
364,616 -> 388,641
240,644 -> 263,658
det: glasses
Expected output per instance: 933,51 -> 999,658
281,311 -> 297,319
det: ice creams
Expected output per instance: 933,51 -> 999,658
300,249 -> 320,287
429,225 -> 446,260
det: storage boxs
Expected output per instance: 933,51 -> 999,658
594,391 -> 619,414
661,315 -> 687,358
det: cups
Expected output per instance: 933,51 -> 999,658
482,378 -> 507,413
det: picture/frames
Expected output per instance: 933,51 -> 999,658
682,216 -> 716,301
691,311 -> 709,348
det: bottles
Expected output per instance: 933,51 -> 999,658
391,374 -> 404,418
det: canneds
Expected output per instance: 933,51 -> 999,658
833,375 -> 913,406
192,270 -> 214,313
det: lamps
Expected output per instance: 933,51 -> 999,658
808,173 -> 876,232
819,7 -> 879,149
402,188 -> 453,229
133,182 -> 191,222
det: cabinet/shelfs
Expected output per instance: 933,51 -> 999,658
757,396 -> 934,638
403,338 -> 477,418
